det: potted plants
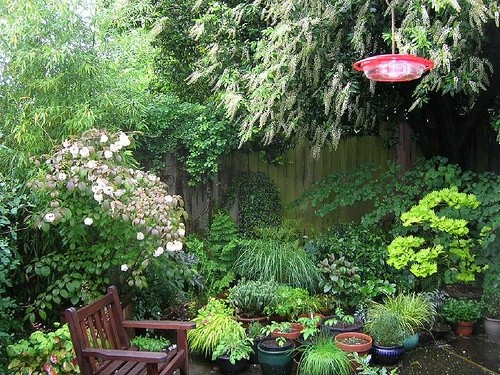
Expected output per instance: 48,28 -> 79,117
187,186 -> 500,375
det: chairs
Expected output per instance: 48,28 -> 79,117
65,284 -> 196,375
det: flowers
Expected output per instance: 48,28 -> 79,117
29,128 -> 187,291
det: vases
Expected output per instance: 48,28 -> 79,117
95,291 -> 136,339
